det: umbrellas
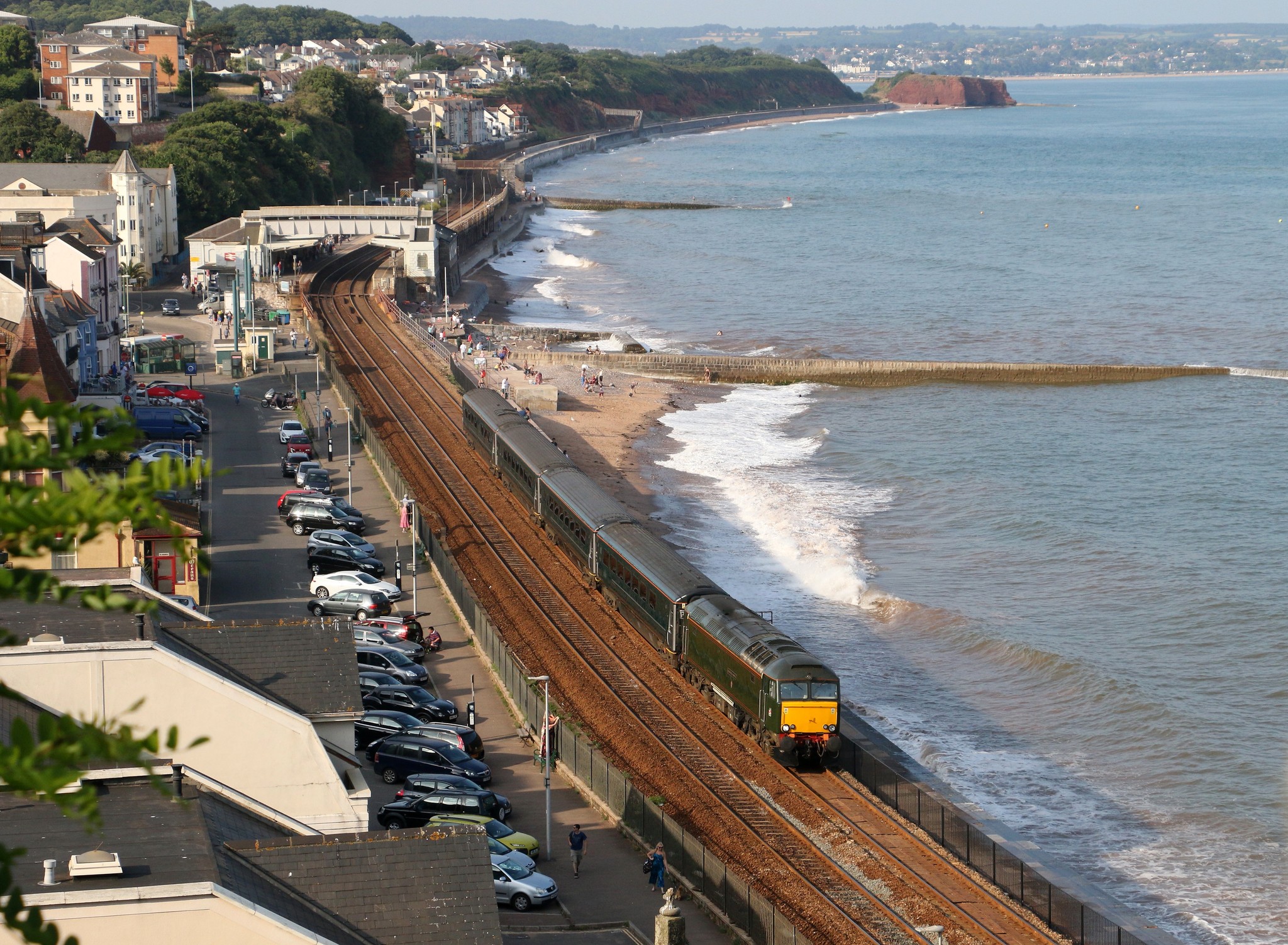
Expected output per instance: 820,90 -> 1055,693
142,387 -> 206,400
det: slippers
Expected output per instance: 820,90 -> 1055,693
651,889 -> 655,891
662,892 -> 665,894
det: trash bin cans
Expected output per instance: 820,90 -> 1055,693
276,309 -> 288,324
279,311 -> 290,324
265,310 -> 276,321
262,309 -> 267,321
269,311 -> 279,321
301,390 -> 307,399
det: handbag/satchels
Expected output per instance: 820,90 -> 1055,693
643,857 -> 653,874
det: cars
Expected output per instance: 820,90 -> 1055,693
195,287 -> 225,314
160,299 -> 180,316
118,333 -> 429,665
489,853 -> 558,913
487,836 -> 537,872
358,672 -> 403,702
355,710 -> 425,750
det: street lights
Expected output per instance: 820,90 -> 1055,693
293,255 -> 297,286
319,177 -> 413,220
245,300 -> 256,373
265,225 -> 273,283
526,676 -> 551,862
400,499 -> 417,629
338,408 -> 352,507
443,193 -> 448,227
308,353 -> 320,441
121,251 -> 146,314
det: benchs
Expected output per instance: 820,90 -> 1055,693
534,748 -> 557,774
412,541 -> 425,559
516,721 -> 531,747
351,431 -> 361,445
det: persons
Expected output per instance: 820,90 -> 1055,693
568,824 -> 588,878
181,273 -> 203,299
400,494 -> 411,533
232,383 -> 242,405
704,366 -> 710,384
525,185 -> 540,203
290,328 -> 298,349
102,361 -> 131,393
425,626 -> 442,651
204,306 -> 233,339
304,336 -> 311,356
149,397 -> 196,407
392,293 -> 640,458
647,842 -> 668,894
322,406 -> 333,438
692,196 -> 695,203
717,330 -> 722,335
272,233 -> 352,278
520,147 -> 526,157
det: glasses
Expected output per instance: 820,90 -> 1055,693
573,827 -> 576,829
658,846 -> 663,849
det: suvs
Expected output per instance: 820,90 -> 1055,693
374,736 -> 492,785
355,646 -> 429,686
365,721 -> 485,763
363,684 -> 459,724
377,785 -> 504,831
424,814 -> 540,857
393,773 -> 512,816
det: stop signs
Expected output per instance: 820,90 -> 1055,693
120,352 -> 128,362
161,334 -> 167,341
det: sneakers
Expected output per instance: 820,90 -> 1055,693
574,873 -> 578,878
576,868 -> 579,873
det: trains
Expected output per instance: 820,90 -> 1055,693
461,387 -> 841,774
